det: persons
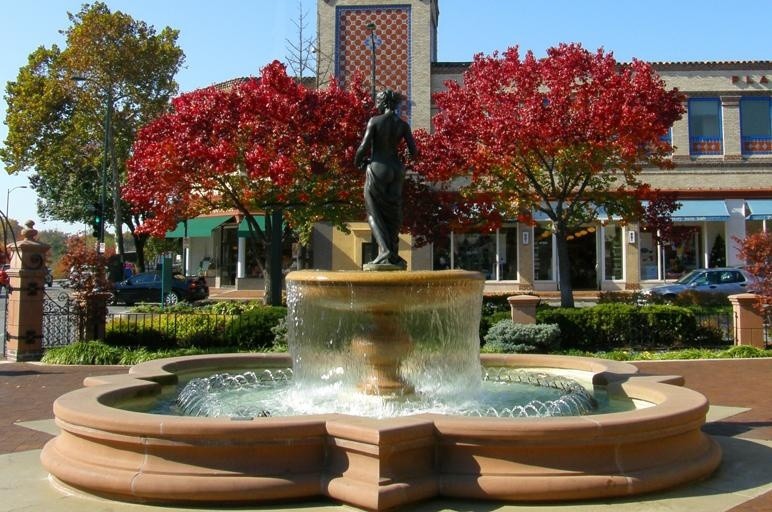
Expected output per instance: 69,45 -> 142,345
355,89 -> 417,265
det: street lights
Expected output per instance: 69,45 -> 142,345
68,74 -> 114,270
4,184 -> 27,264
365,21 -> 379,103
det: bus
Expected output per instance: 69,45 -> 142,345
122,251 -> 182,275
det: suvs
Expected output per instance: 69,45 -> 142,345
69,264 -> 109,284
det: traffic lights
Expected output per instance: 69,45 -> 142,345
90,201 -> 101,237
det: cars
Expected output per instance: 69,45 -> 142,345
637,267 -> 769,308
92,270 -> 210,307
662,264 -> 696,280
0,265 -> 10,293
44,271 -> 54,287
